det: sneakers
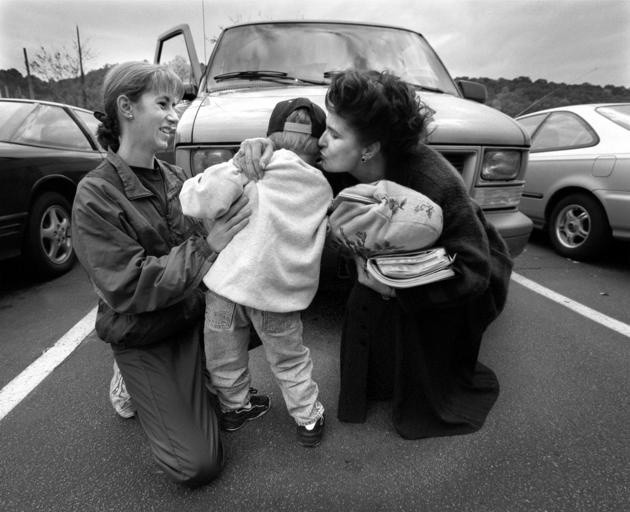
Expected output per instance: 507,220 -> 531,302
205,373 -> 271,432
109,360 -> 135,418
296,414 -> 325,447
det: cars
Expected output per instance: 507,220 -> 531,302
509,101 -> 630,263
0,96 -> 124,280
150,16 -> 535,285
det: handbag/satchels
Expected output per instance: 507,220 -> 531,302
327,180 -> 444,259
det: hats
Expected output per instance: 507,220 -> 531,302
267,98 -> 326,138
